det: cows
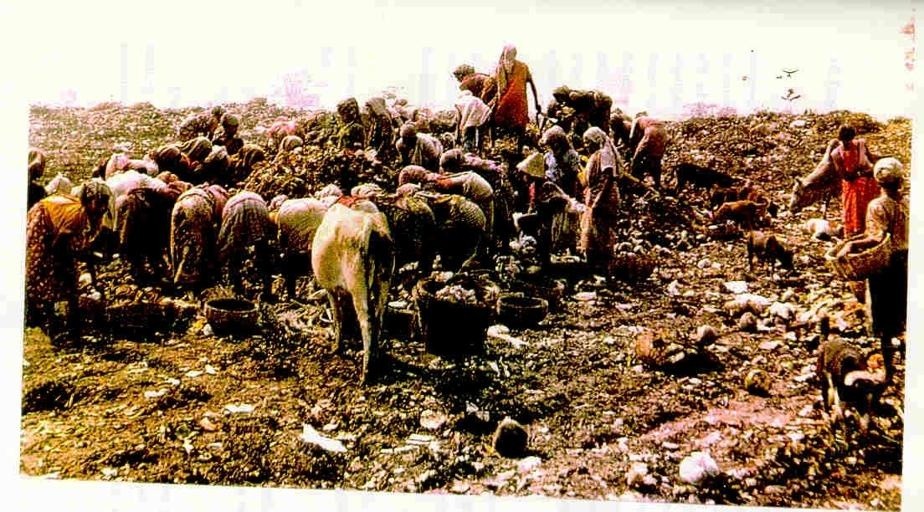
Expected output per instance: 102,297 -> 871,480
742,230 -> 795,278
309,198 -> 396,383
788,137 -> 852,219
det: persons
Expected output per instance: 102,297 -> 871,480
490,45 -> 541,144
833,150 -> 911,384
831,125 -> 881,241
455,64 -> 496,140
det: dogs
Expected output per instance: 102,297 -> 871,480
670,163 -> 734,191
815,315 -> 877,451
708,196 -> 772,226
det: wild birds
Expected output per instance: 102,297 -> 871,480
781,86 -> 801,103
783,64 -> 797,78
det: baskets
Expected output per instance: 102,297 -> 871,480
616,251 -> 659,283
77,292 -> 105,321
512,273 -> 567,312
417,272 -> 500,363
204,295 -> 260,339
108,301 -> 165,337
824,231 -> 893,282
495,296 -> 549,329
